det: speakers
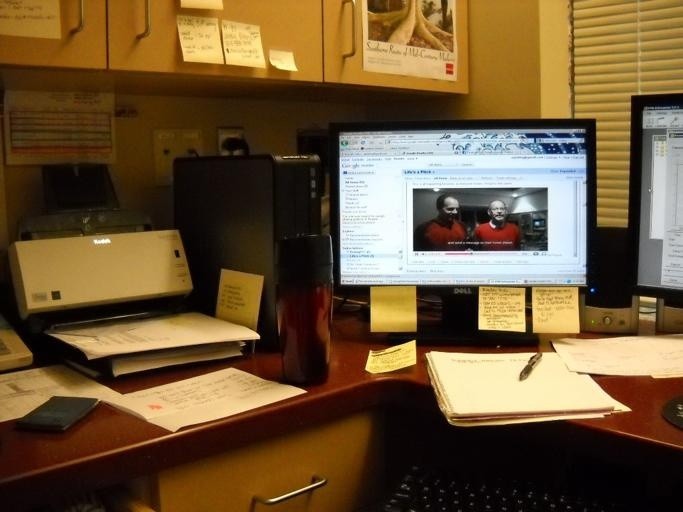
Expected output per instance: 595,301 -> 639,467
655,297 -> 683,334
40,162 -> 119,214
579,226 -> 640,336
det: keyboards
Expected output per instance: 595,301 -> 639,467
381,457 -> 628,511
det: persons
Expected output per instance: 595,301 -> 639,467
414,193 -> 521,249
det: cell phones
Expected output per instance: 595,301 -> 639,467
15,395 -> 101,431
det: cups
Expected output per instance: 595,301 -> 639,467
276,232 -> 334,384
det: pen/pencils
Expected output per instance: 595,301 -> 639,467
520,352 -> 543,381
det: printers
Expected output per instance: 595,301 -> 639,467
8,229 -> 246,381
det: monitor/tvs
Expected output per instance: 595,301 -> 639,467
329,116 -> 598,348
627,92 -> 683,298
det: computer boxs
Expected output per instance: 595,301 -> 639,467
171,154 -> 323,351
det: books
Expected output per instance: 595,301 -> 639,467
0,267 -> 307,438
425,351 -> 616,428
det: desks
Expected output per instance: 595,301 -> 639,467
0,321 -> 683,512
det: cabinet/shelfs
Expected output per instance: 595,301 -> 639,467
323,0 -> 472,94
2,0 -> 325,88
